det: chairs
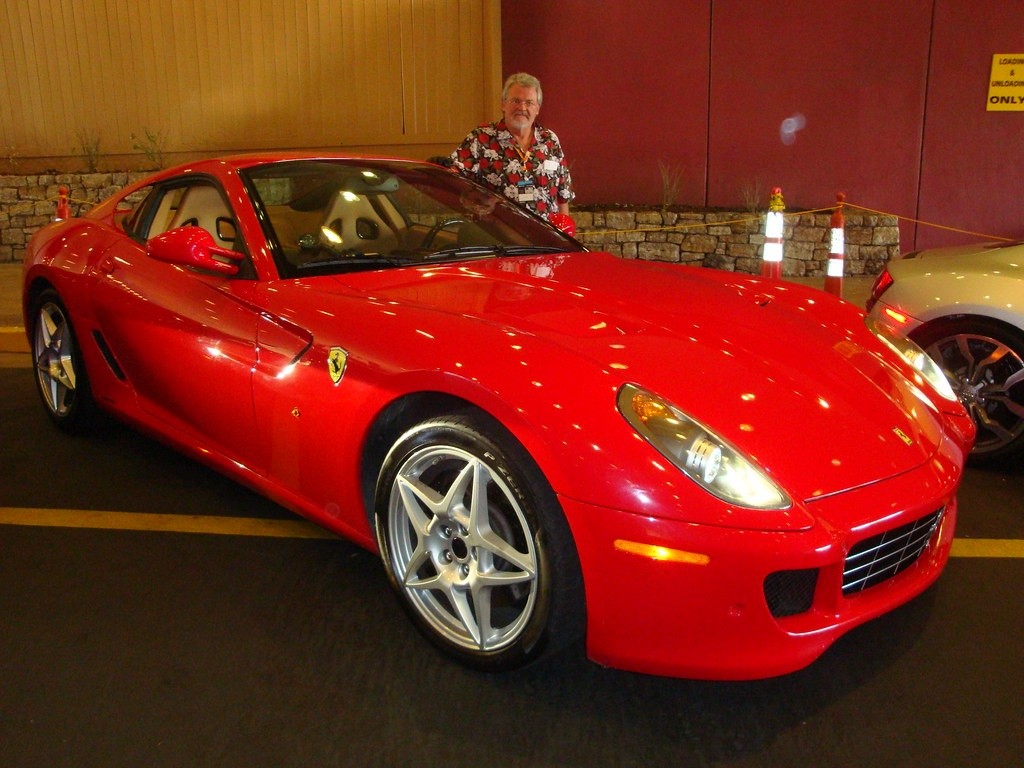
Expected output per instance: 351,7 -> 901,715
315,189 -> 399,259
166,187 -> 236,262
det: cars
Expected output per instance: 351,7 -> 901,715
865,240 -> 1023,466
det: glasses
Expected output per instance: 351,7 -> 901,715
508,97 -> 535,110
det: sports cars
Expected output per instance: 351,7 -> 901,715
23,149 -> 978,683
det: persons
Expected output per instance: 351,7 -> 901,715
452,73 -> 576,246
